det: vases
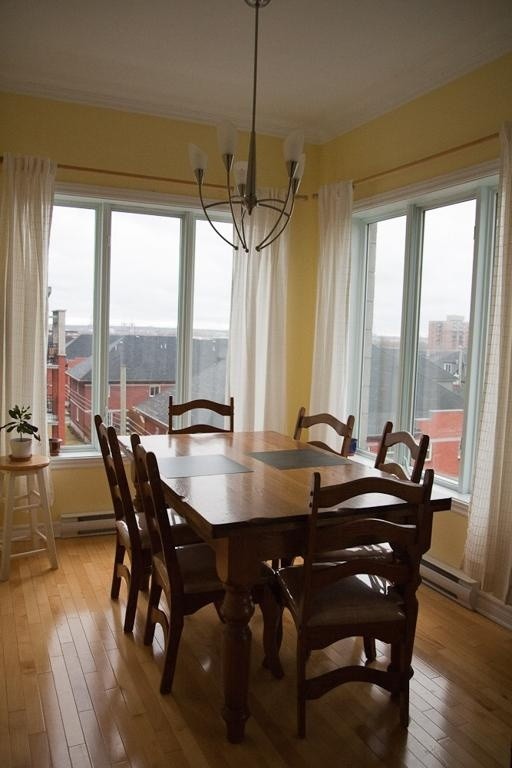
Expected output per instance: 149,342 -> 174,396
49,438 -> 62,456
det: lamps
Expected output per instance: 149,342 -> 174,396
189,1 -> 307,252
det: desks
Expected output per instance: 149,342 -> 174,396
117,431 -> 452,745
0,456 -> 58,582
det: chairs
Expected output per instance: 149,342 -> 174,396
131,435 -> 276,695
275,469 -> 435,735
293,408 -> 356,457
168,396 -> 234,435
303,421 -> 429,565
96,414 -> 206,632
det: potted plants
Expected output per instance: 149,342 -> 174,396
0,404 -> 40,462
